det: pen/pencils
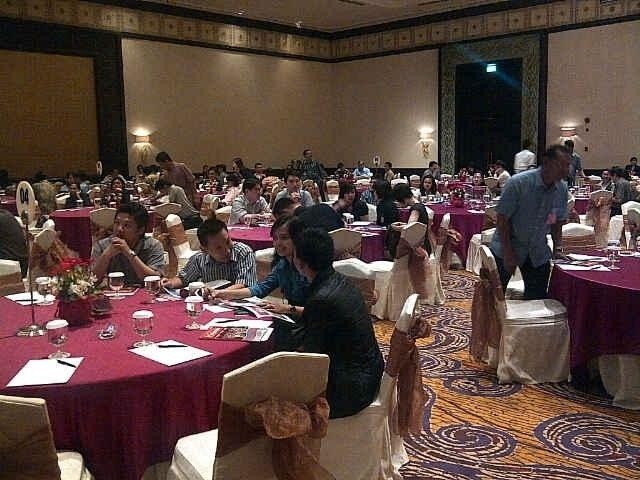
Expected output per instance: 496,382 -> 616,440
158,345 -> 189,348
216,318 -> 242,323
16,300 -> 38,301
57,360 -> 76,367
591,266 -> 601,270
584,258 -> 604,262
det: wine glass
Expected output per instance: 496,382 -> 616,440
244,216 -> 251,229
418,192 -> 490,210
341,212 -> 354,230
75,195 -> 151,214
607,236 -> 640,271
34,271 -> 208,360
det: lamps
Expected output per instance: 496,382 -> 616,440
560,126 -> 577,141
416,127 -> 435,159
131,131 -> 152,163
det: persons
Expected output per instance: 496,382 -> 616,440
200,215 -> 384,421
1,140 -> 639,299
